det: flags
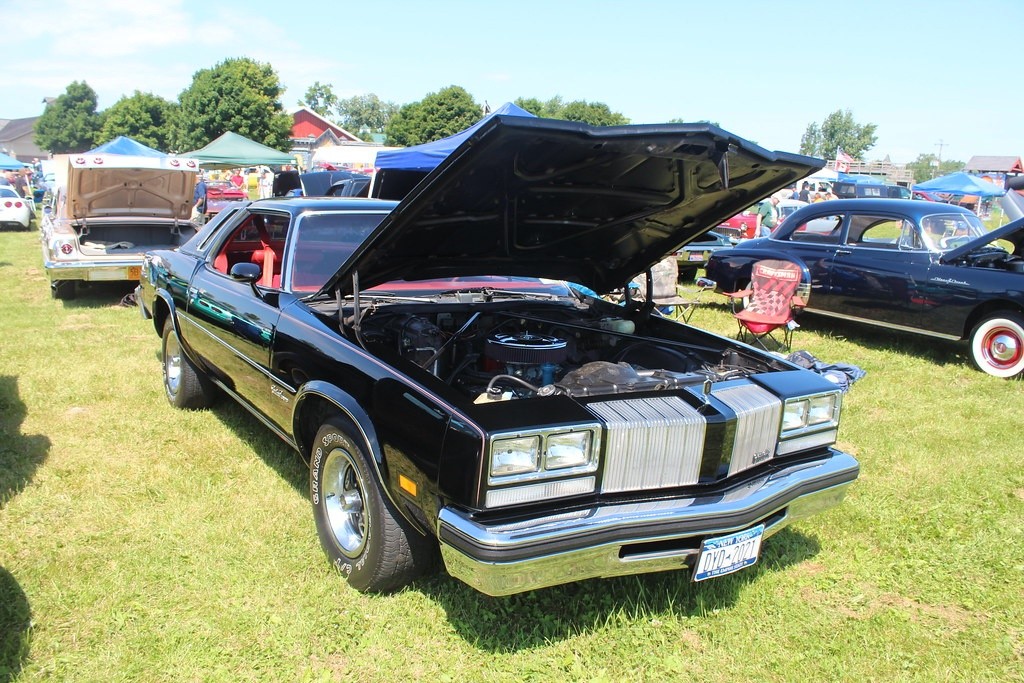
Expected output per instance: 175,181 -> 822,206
835,161 -> 850,173
837,148 -> 853,162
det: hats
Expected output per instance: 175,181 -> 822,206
772,192 -> 783,202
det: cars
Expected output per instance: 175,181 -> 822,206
0,186 -> 31,231
706,197 -> 1024,377
41,154 -> 201,299
299,171 -> 370,197
756,199 -> 838,233
140,113 -> 862,596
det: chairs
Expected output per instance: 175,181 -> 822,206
251,250 -> 295,294
724,260 -> 803,351
634,258 -> 715,324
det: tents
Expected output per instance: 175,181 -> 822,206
0,153 -> 36,211
910,171 -> 1006,227
367,102 -> 535,198
84,136 -> 167,157
177,130 -> 306,197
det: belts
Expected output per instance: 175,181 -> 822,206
760,223 -> 765,226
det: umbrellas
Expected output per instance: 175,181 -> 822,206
811,168 -> 882,185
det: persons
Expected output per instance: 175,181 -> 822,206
826,192 -> 840,220
754,192 -> 782,237
791,186 -> 798,199
218,167 -> 244,188
0,175 -> 9,186
799,181 -> 811,203
14,170 -> 26,196
191,175 -> 207,221
34,157 -> 43,189
813,193 -> 824,203
